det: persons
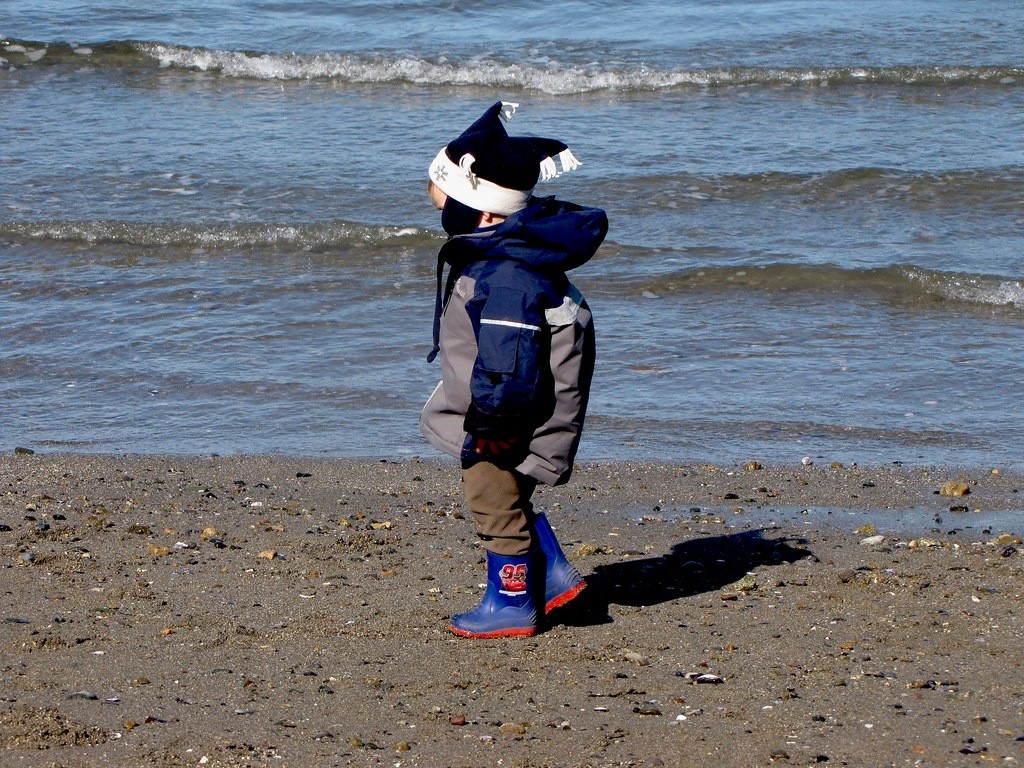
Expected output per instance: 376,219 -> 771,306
418,133 -> 597,639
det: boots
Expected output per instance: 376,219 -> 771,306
448,547 -> 538,639
527,511 -> 588,616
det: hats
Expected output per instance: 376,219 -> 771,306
427,102 -> 569,215
